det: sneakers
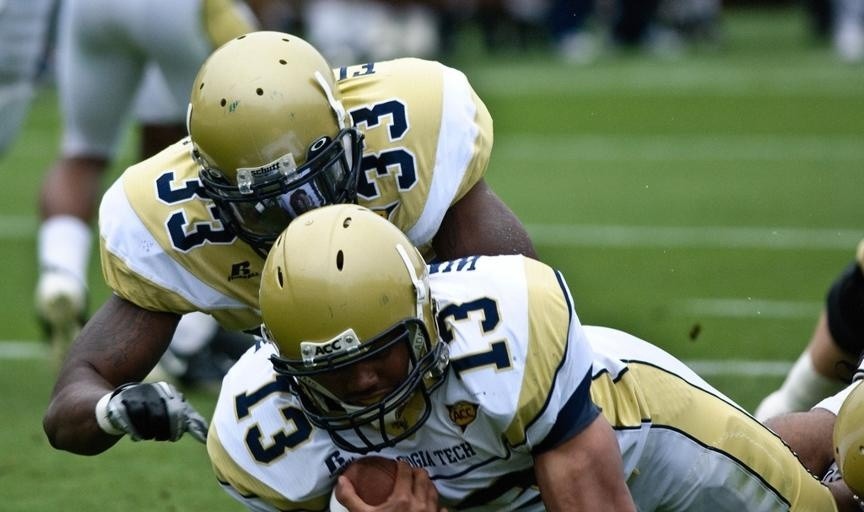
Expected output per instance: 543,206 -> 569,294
160,312 -> 256,385
823,462 -> 842,483
34,272 -> 91,357
852,352 -> 864,382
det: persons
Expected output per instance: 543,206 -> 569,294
755,240 -> 862,509
207,205 -> 837,510
44,31 -> 536,456
135,64 -> 187,158
834,383 -> 862,496
37,1 -> 257,365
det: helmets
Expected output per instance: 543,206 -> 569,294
188,32 -> 352,187
832,378 -> 864,501
259,204 -> 438,387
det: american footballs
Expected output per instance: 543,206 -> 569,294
325,455 -> 442,512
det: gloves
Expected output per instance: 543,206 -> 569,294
95,381 -> 209,444
